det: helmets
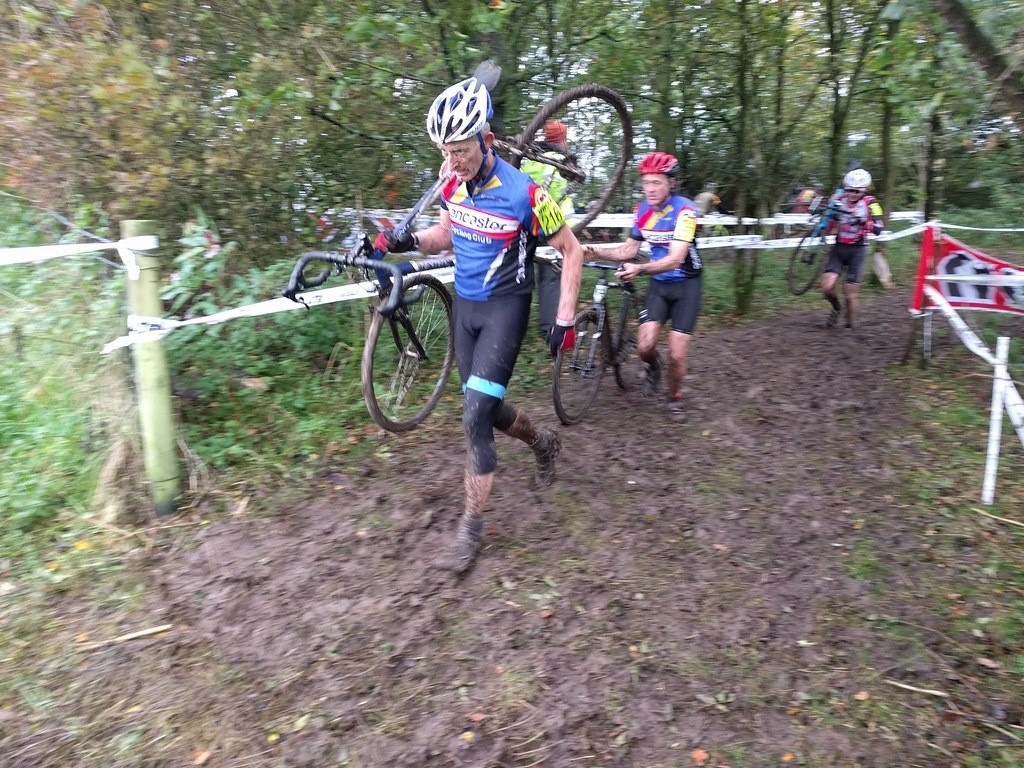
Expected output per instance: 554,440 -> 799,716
843,169 -> 873,191
703,181 -> 720,189
426,78 -> 495,140
638,153 -> 681,177
542,121 -> 566,141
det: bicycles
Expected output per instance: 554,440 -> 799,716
281,53 -> 634,435
778,185 -> 866,296
547,257 -> 649,427
690,209 -> 742,263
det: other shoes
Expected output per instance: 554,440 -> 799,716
534,428 -> 561,486
641,361 -> 689,422
437,531 -> 480,572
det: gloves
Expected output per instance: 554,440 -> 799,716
372,232 -> 414,254
547,320 -> 575,354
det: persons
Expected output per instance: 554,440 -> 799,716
367,77 -> 884,576
